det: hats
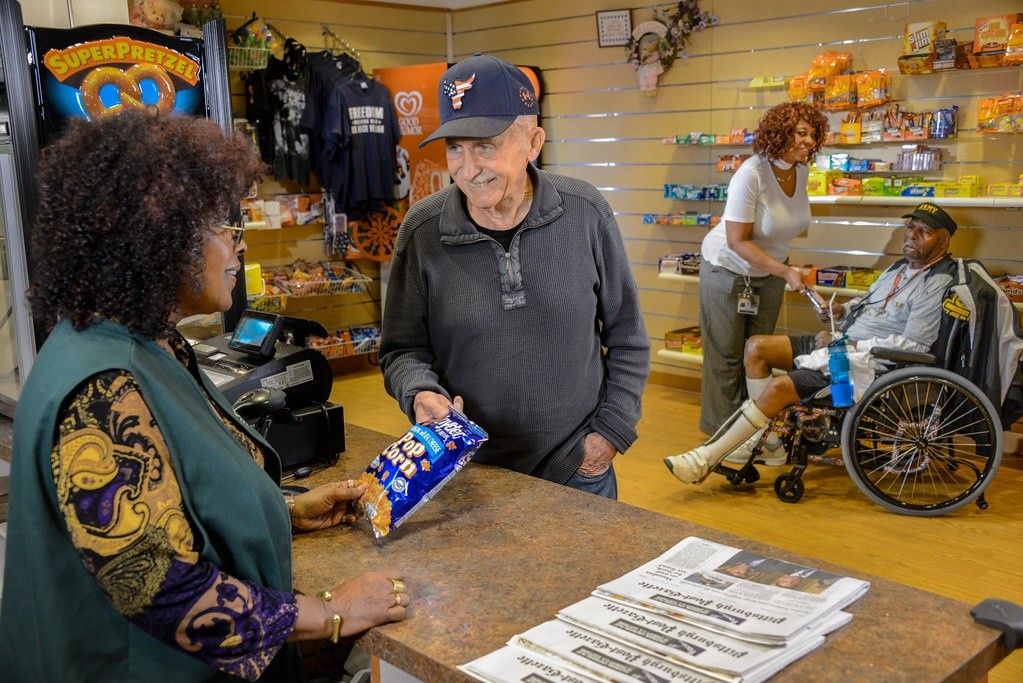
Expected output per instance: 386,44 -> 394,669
901,202 -> 957,237
419,51 -> 540,148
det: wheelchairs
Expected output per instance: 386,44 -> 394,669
691,257 -> 1023,516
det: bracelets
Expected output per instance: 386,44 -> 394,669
317,589 -> 341,644
288,493 -> 296,521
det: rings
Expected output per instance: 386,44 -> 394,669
347,478 -> 354,488
342,514 -> 348,522
394,594 -> 401,605
391,579 -> 404,593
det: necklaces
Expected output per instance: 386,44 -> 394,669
775,169 -> 792,181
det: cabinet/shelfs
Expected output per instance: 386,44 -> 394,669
658,46 -> 1023,456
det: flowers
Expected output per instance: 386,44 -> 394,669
624,0 -> 718,71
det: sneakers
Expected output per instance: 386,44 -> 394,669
663,445 -> 711,483
724,439 -> 788,467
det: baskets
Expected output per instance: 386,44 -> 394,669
271,267 -> 372,298
304,336 -> 382,361
228,47 -> 272,71
247,282 -> 291,314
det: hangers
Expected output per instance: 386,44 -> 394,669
282,29 -> 371,83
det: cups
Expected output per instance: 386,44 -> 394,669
240,200 -> 282,228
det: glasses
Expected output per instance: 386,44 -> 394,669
213,218 -> 246,245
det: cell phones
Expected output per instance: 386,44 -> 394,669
807,289 -> 830,313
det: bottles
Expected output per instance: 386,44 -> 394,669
827,335 -> 854,407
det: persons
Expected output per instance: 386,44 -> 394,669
699,101 -> 831,437
662,202 -> 959,485
0,115 -> 412,683
375,53 -> 650,501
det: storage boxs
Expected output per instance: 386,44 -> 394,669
660,252 -> 700,275
664,325 -> 702,356
793,265 -> 886,290
898,13 -> 1019,75
989,273 -> 1023,302
840,120 -> 884,145
806,167 -> 1023,197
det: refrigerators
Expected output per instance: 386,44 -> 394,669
372,61 -> 545,339
